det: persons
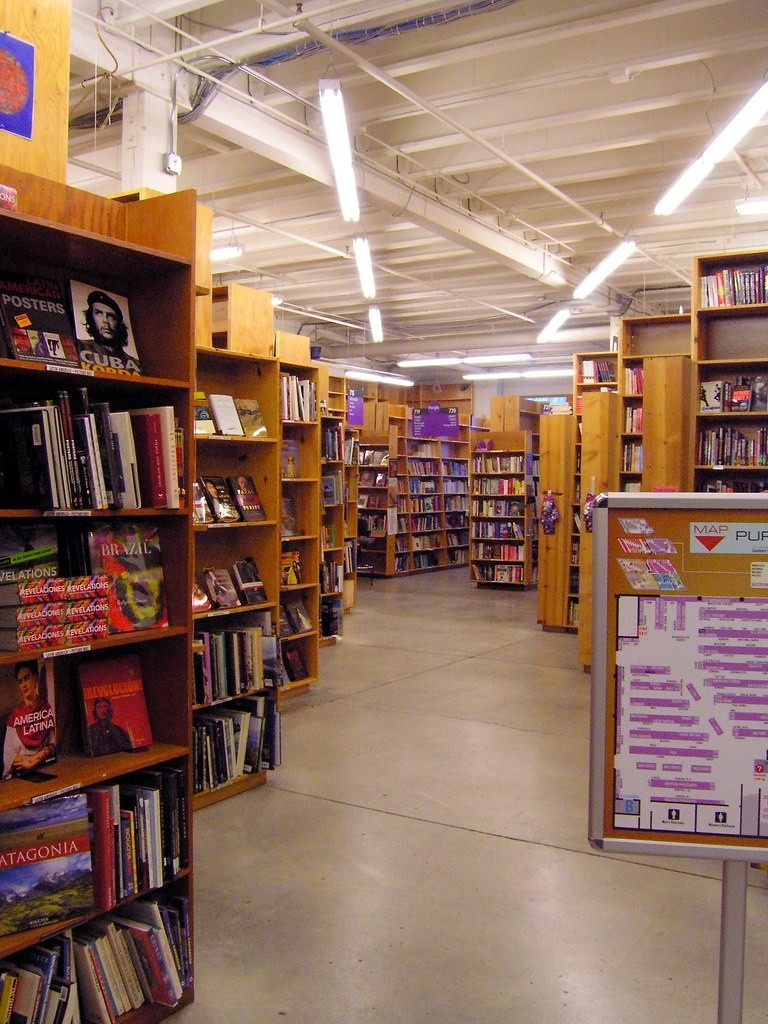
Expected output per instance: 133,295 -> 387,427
77,291 -> 140,368
4,659 -> 55,775
89,698 -> 131,756
238,476 -> 248,490
206,480 -> 218,498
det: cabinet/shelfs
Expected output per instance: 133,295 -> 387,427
0,165 -> 768,1024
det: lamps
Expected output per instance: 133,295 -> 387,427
536,309 -> 570,344
465,354 -> 532,364
397,358 -> 464,367
319,78 -> 361,220
344,370 -> 414,387
368,304 -> 383,343
654,80 -> 768,215
572,236 -> 637,300
463,373 -> 525,380
351,233 -> 376,299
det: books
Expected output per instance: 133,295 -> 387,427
472,454 -> 524,582
394,461 -> 470,572
193,376 -> 316,794
576,484 -> 580,502
320,426 -> 389,636
623,367 -> 644,492
700,374 -> 768,493
583,360 -> 612,382
577,453 -> 580,472
701,265 -> 768,305
0,279 -> 193,1024
570,572 -> 579,595
572,543 -> 579,563
573,513 -> 580,529
569,601 -> 579,626
540,403 -> 573,415
532,459 -> 539,496
576,395 -> 582,414
